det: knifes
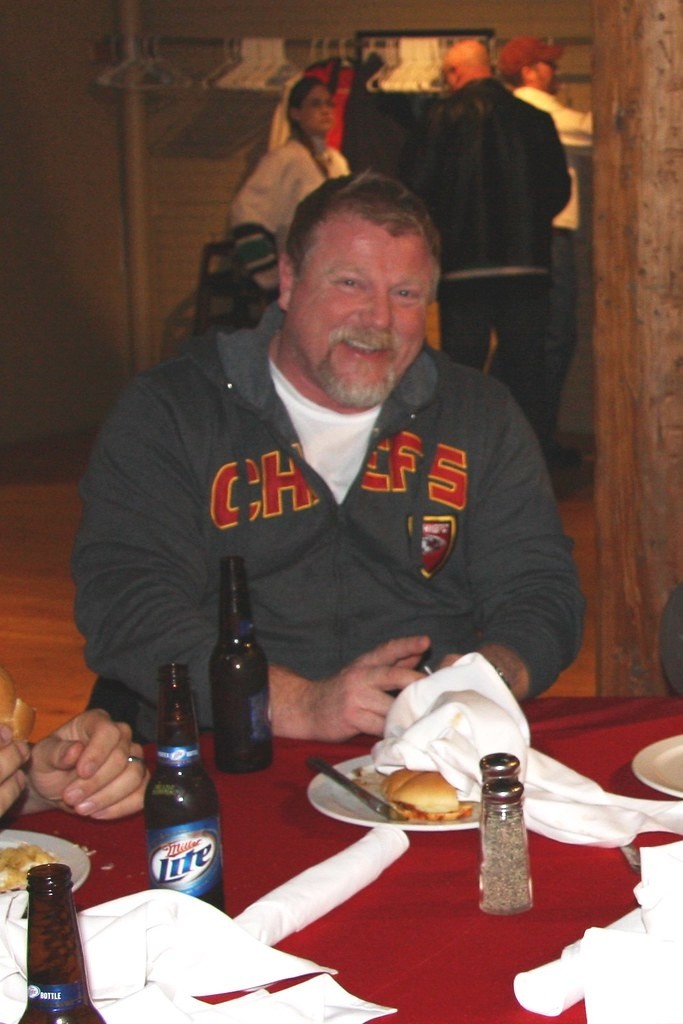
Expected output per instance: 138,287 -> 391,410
306,757 -> 409,820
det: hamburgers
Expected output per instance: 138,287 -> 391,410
383,767 -> 472,821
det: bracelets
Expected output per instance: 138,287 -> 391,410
491,661 -> 510,689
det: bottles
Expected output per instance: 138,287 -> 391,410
209,554 -> 273,774
485,757 -> 522,779
143,663 -> 225,917
19,863 -> 107,1024
480,777 -> 532,913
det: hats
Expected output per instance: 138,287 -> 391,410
498,37 -> 563,79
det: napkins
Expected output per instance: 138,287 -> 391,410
371,652 -> 683,849
514,831 -> 683,1024
0,826 -> 409,1024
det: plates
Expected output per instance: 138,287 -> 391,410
630,734 -> 683,799
0,827 -> 91,894
309,754 -> 482,830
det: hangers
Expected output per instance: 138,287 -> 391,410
94,32 -> 352,95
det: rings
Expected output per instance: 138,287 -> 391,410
129,756 -> 148,764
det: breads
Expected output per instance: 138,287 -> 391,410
0,662 -> 36,742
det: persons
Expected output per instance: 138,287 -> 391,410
68,169 -> 588,739
231,75 -> 354,290
0,667 -> 152,820
497,33 -> 592,477
402,39 -> 573,494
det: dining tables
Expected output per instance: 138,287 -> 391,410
1,697 -> 683,1024
